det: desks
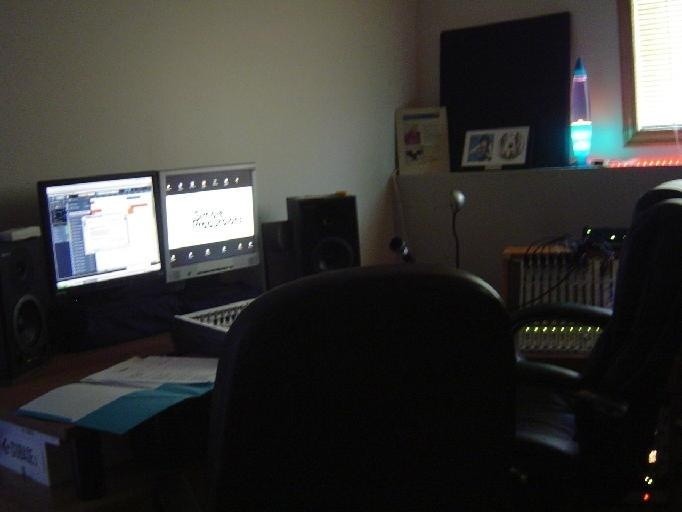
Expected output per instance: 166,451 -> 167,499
0,331 -> 218,512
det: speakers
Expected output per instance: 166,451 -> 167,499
286,195 -> 361,280
0,235 -> 59,380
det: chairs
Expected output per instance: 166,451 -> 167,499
515,179 -> 681,512
60,261 -> 515,512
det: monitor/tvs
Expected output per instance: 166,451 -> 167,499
160,162 -> 264,300
38,171 -> 166,309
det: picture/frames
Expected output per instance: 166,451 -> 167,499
458,122 -> 535,172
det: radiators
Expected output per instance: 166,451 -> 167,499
512,256 -> 619,352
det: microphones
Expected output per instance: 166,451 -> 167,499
390,237 -> 410,262
